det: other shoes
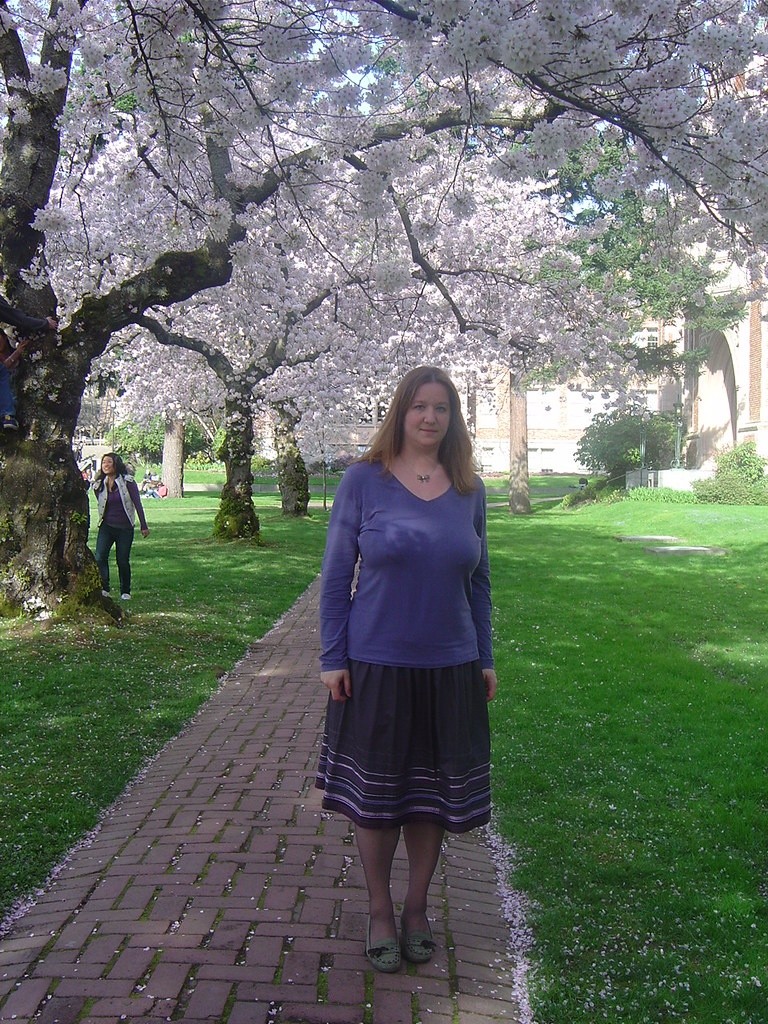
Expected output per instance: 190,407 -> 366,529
3,418 -> 19,430
121,592 -> 131,600
401,910 -> 436,963
366,914 -> 402,972
102,589 -> 109,598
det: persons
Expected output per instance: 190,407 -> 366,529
315,365 -> 497,973
92,452 -> 151,601
140,469 -> 168,500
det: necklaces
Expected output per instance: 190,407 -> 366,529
396,452 -> 442,482
